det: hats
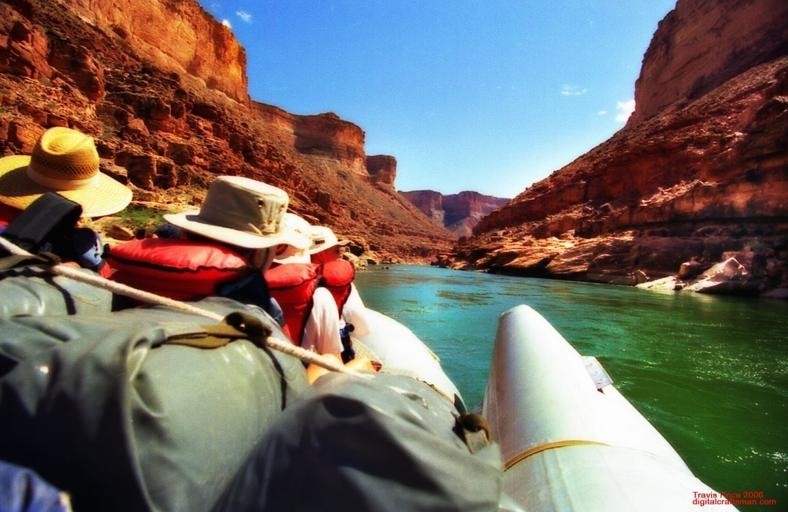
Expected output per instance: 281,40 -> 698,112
0,125 -> 136,220
163,176 -> 353,266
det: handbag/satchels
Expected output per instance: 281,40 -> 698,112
1,265 -> 506,511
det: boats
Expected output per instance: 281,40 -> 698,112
339,282 -> 737,512
